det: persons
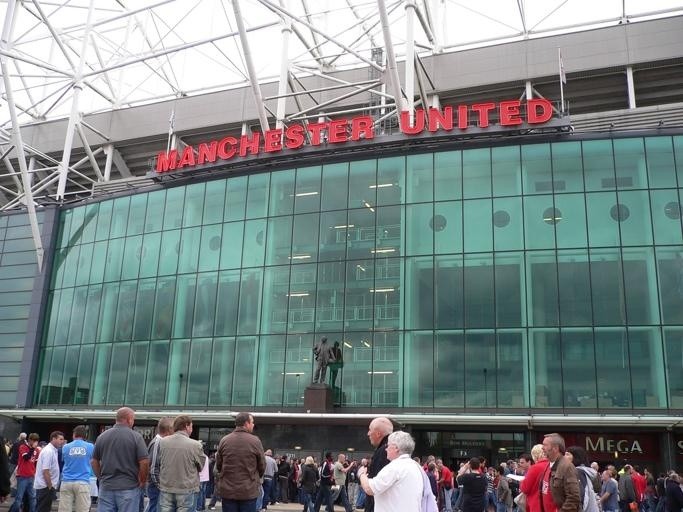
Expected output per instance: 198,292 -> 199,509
1,425 -> 682,512
365,415 -> 394,512
312,336 -> 336,385
159,416 -> 205,512
142,417 -> 175,512
328,341 -> 343,386
215,412 -> 266,512
90,407 -> 149,512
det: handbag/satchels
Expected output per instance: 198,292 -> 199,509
422,489 -> 439,512
